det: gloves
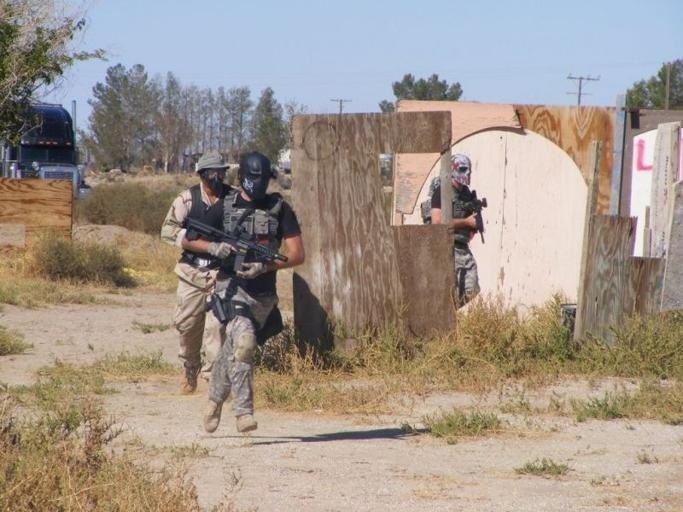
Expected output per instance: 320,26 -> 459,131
204,241 -> 238,259
236,261 -> 277,280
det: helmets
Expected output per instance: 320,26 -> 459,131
450,153 -> 472,187
238,152 -> 271,202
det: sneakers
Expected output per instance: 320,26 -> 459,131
204,398 -> 222,433
180,384 -> 198,396
237,412 -> 258,433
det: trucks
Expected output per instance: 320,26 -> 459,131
0,102 -> 82,204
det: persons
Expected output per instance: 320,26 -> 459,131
176,151 -> 307,435
418,148 -> 488,317
159,150 -> 244,397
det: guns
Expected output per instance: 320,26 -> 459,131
180,216 -> 288,271
204,292 -> 227,324
471,190 -> 486,243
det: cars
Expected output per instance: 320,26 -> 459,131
278,148 -> 293,173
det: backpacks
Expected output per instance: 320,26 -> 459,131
421,199 -> 432,226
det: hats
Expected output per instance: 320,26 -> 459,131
197,148 -> 230,173
